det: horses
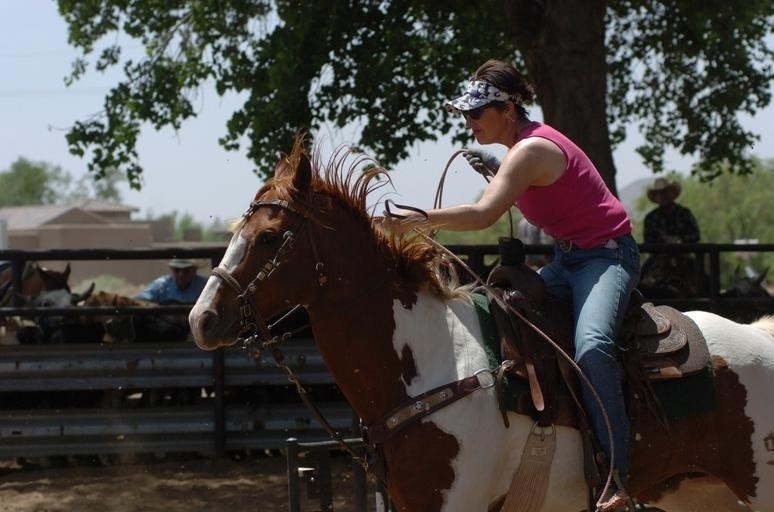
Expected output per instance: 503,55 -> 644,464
2,260 -> 184,465
185,139 -> 774,511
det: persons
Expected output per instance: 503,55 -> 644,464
515,216 -> 557,270
381,58 -> 641,512
129,257 -> 211,345
640,178 -> 702,279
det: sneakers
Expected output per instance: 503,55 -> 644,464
593,480 -> 628,512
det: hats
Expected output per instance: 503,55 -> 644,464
168,259 -> 197,268
445,81 -> 522,113
647,179 -> 681,202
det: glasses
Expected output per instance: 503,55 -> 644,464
462,102 -> 497,120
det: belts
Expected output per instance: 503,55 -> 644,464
556,238 -> 619,253
